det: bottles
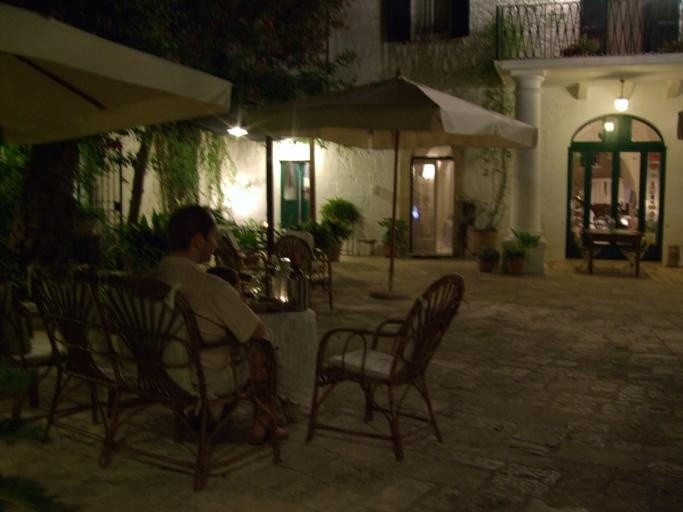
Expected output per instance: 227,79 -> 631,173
275,257 -> 304,305
112,247 -> 129,275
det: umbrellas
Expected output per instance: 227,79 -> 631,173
0,2 -> 232,147
240,73 -> 540,289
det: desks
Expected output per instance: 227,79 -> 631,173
584,228 -> 645,276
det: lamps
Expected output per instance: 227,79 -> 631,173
615,80 -> 630,112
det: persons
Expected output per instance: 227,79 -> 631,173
131,203 -> 291,443
568,196 -> 638,229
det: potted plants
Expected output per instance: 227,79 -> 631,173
320,198 -> 539,275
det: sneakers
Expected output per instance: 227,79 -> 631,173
247,423 -> 289,443
